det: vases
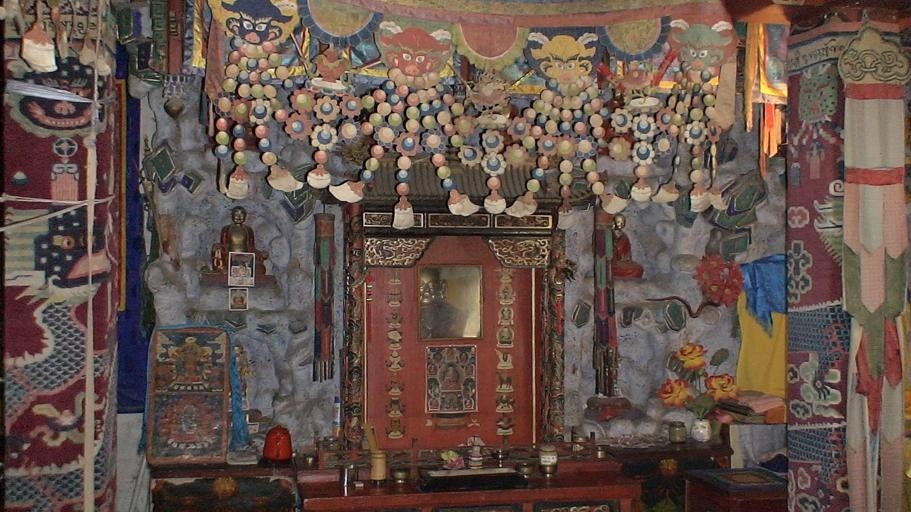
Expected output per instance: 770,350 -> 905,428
690,418 -> 712,444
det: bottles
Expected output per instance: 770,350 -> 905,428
263,424 -> 291,460
670,421 -> 687,443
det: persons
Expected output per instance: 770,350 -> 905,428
208,204 -> 269,276
418,268 -> 462,339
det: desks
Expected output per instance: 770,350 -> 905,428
298,472 -> 642,512
684,469 -> 787,512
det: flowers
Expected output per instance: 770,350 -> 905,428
693,254 -> 743,307
658,343 -> 739,421
